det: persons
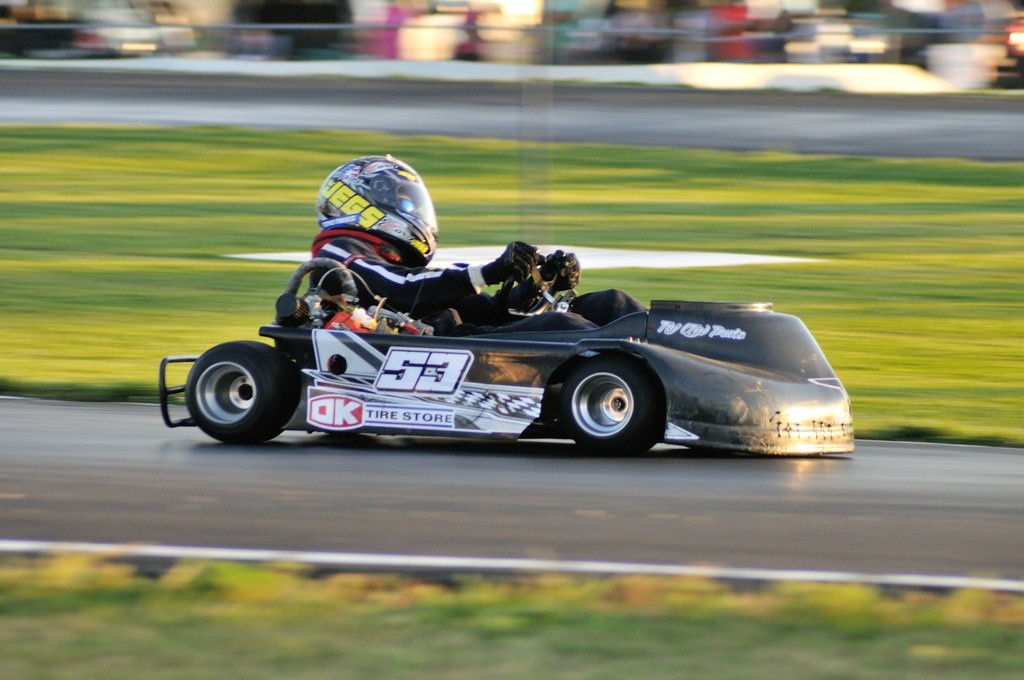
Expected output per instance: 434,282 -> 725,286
310,155 -> 650,337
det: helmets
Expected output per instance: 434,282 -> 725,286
315,154 -> 439,267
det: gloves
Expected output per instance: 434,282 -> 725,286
481,240 -> 547,287
540,250 -> 580,292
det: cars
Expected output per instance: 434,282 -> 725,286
0,0 -> 172,61
